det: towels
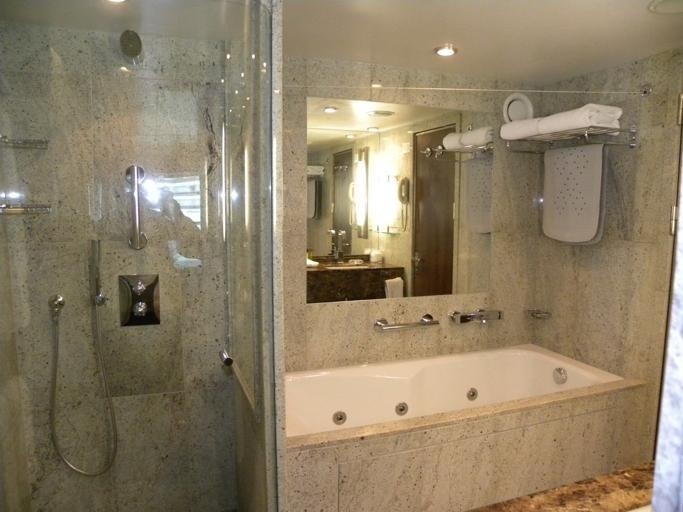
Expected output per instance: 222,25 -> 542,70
500,119 -> 555,143
307,259 -> 319,267
385,278 -> 403,298
542,144 -> 606,246
464,157 -> 492,234
307,180 -> 316,219
537,103 -> 622,137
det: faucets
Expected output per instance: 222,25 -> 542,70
330,228 -> 347,265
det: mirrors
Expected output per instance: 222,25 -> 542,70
306,96 -> 493,303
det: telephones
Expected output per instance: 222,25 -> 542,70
397,178 -> 411,232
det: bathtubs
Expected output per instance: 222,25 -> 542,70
285,343 -> 625,439
